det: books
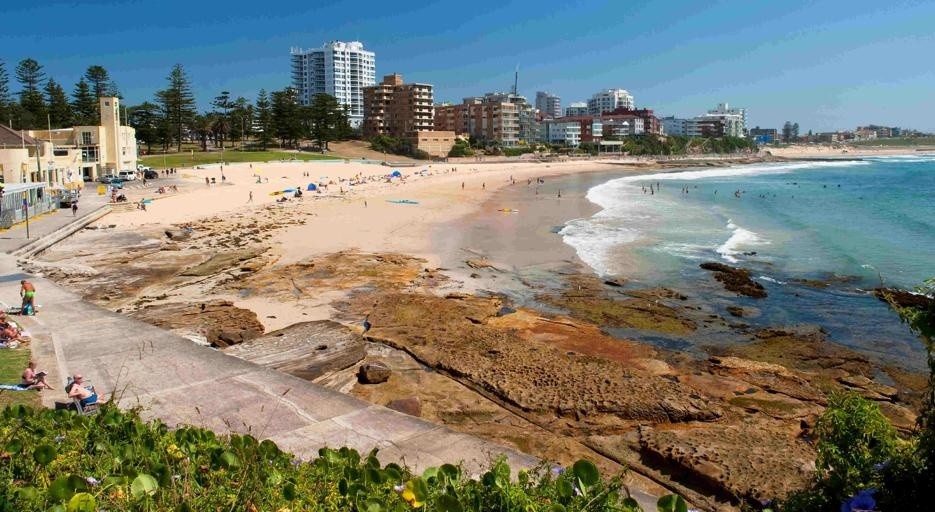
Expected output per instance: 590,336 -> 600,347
35,371 -> 48,376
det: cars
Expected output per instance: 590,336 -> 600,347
144,171 -> 158,179
100,175 -> 123,184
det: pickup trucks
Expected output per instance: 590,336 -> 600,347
136,164 -> 151,172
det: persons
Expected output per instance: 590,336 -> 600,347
271,166 -> 464,200
70,198 -> 77,218
16,279 -> 35,316
0,315 -> 14,331
246,191 -> 253,203
110,173 -> 168,211
22,359 -> 57,391
68,373 -> 108,407
0,323 -> 31,344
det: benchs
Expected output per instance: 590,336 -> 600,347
70,380 -> 103,416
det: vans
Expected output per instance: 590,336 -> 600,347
119,170 -> 137,181
